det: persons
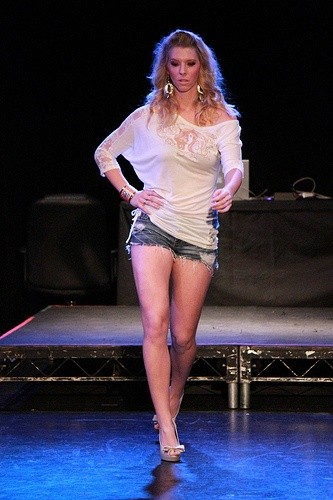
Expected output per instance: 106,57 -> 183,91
93,29 -> 244,462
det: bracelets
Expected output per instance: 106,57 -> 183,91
118,183 -> 139,203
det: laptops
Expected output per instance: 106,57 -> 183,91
216,159 -> 249,200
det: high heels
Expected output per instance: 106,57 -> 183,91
159,418 -> 185,461
152,385 -> 184,433
